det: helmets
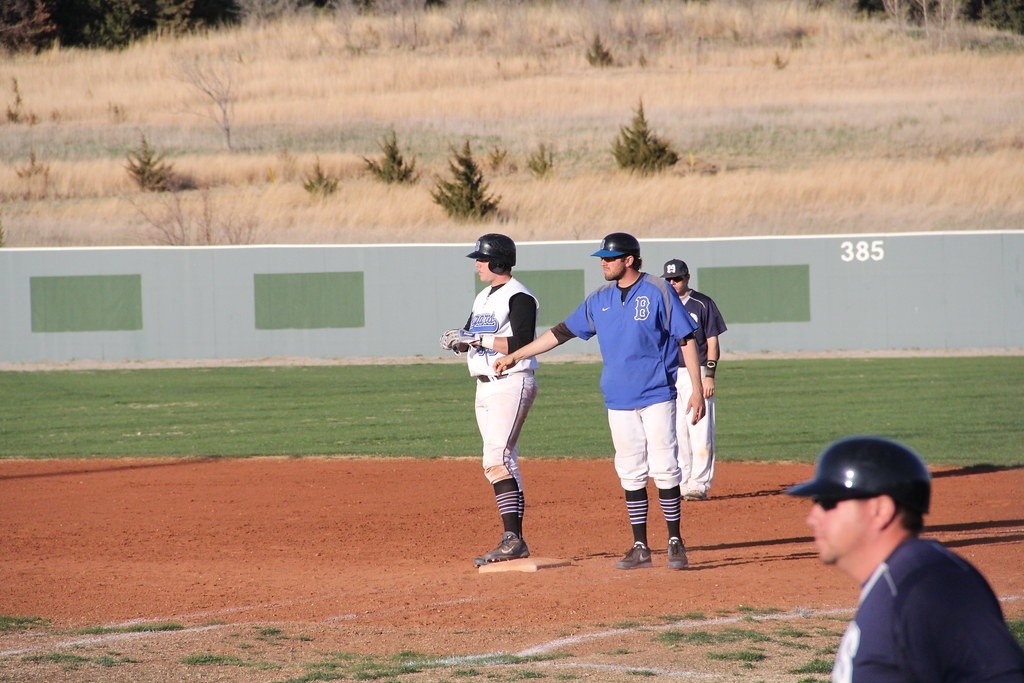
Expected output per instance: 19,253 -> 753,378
590,233 -> 641,257
467,233 -> 516,275
787,436 -> 930,512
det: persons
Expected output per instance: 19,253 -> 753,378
780,437 -> 1024,683
492,231 -> 706,570
440,233 -> 540,568
660,259 -> 729,502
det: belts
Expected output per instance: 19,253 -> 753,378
477,374 -> 507,382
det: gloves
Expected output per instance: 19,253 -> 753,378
441,333 -> 461,356
445,329 -> 480,351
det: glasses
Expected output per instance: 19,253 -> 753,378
665,276 -> 682,282
812,494 -> 838,511
601,255 -> 628,262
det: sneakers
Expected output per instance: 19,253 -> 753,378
667,536 -> 688,568
472,556 -> 485,567
485,532 -> 530,562
616,541 -> 652,568
684,490 -> 708,501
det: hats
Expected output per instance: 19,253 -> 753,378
660,259 -> 688,278
